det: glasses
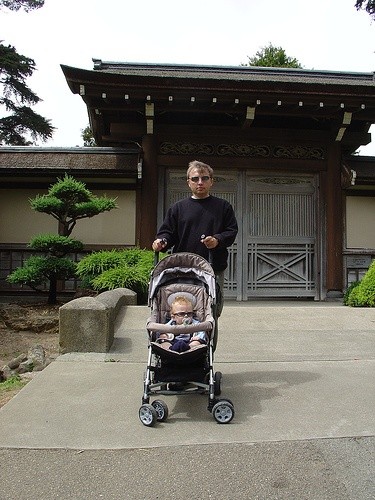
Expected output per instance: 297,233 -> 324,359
188,176 -> 212,181
173,312 -> 195,317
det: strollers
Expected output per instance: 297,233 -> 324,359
138,234 -> 235,427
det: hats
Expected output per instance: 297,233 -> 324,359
167,291 -> 197,309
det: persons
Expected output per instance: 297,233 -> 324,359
156,296 -> 207,353
151,159 -> 238,362
